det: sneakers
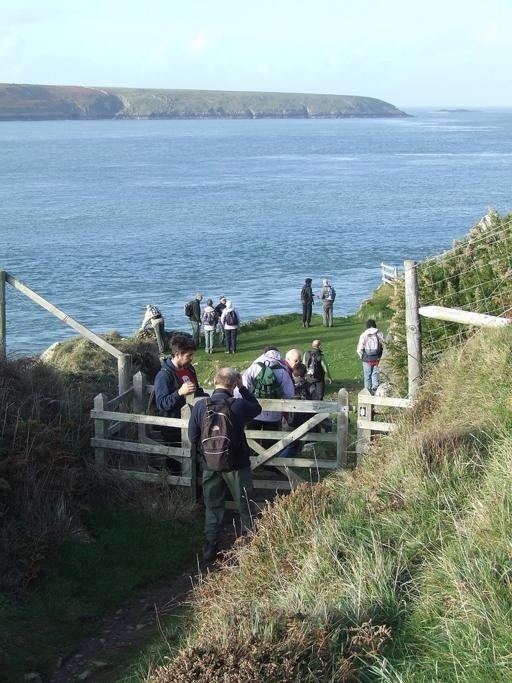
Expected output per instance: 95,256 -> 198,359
303,322 -> 305,328
225,351 -> 230,354
232,351 -> 235,354
202,542 -> 216,561
306,322 -> 311,327
210,350 -> 215,354
204,348 -> 210,353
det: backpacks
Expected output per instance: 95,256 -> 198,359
149,307 -> 161,319
202,312 -> 216,325
364,334 -> 380,356
285,382 -> 314,427
301,287 -> 310,305
145,368 -> 177,431
323,286 -> 335,301
305,353 -> 322,382
226,311 -> 238,325
185,301 -> 199,316
199,396 -> 239,472
249,360 -> 286,398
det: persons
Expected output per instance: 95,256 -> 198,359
187,293 -> 239,354
154,332 -> 211,490
357,318 -> 385,395
278,362 -> 331,457
301,278 -> 315,327
139,305 -> 164,354
302,339 -> 333,399
232,346 -> 295,456
188,368 -> 263,560
317,279 -> 336,326
281,349 -> 302,370
